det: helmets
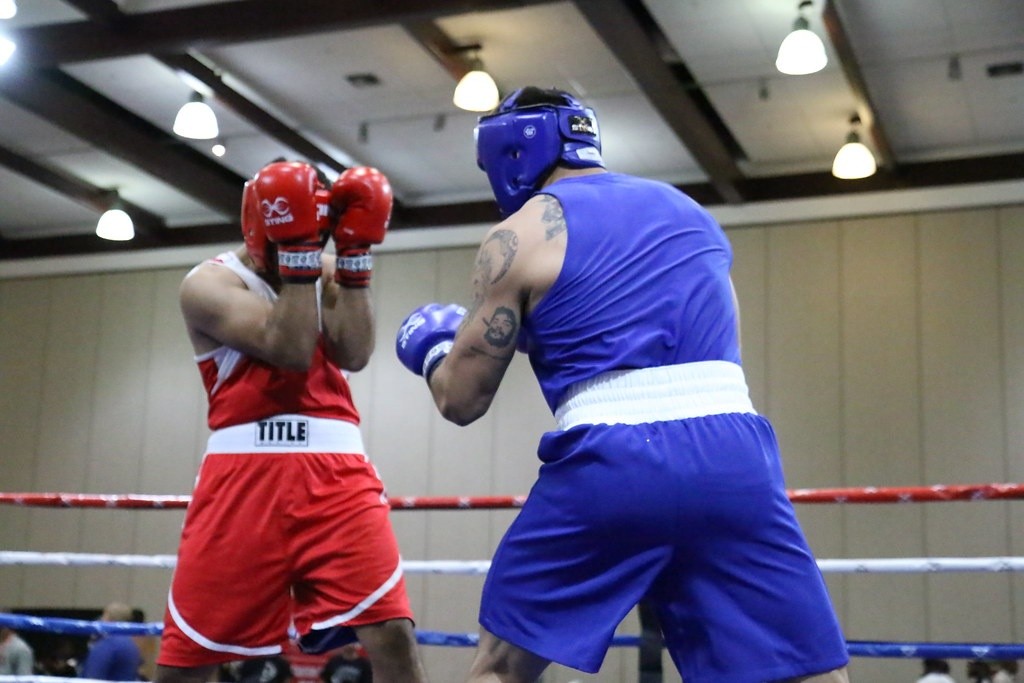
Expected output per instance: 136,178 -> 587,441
474,85 -> 604,215
241,169 -> 334,274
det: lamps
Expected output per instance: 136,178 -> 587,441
775,0 -> 828,75
452,44 -> 500,112
96,188 -> 135,241
172,91 -> 219,140
832,115 -> 877,180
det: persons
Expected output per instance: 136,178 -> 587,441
915,655 -> 1017,683
391,83 -> 853,683
157,167 -> 422,683
75,607 -> 160,682
0,607 -> 35,676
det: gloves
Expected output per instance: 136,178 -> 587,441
327,167 -> 394,288
397,301 -> 471,385
254,164 -> 331,279
